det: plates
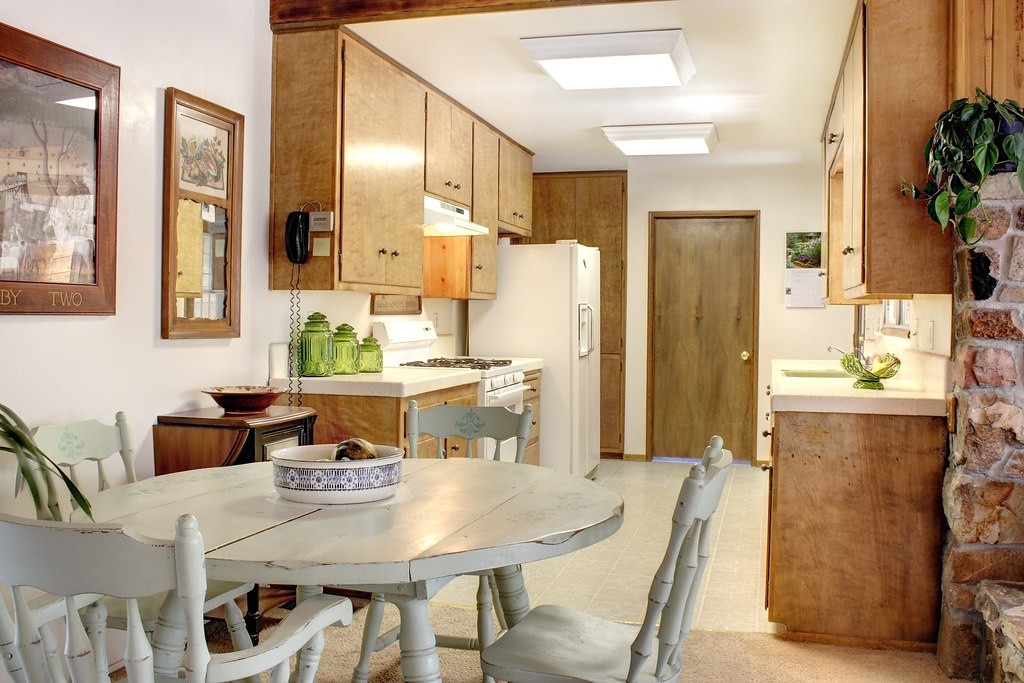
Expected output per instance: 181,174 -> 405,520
841,353 -> 900,390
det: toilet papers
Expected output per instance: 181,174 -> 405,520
269,343 -> 292,378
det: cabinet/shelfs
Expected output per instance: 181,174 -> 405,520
425,88 -> 474,212
763,409 -> 948,650
522,369 -> 544,467
499,135 -> 534,238
511,169 -> 625,454
821,0 -> 951,304
146,405 -> 318,647
269,26 -> 421,296
275,383 -> 480,465
421,124 -> 501,300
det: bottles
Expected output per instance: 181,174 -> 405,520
297,312 -> 382,376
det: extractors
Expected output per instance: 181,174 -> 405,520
423,196 -> 490,235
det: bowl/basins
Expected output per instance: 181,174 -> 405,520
201,386 -> 287,414
270,444 -> 405,504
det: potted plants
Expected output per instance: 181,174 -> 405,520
899,87 -> 1024,245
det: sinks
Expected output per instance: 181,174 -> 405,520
780,370 -> 856,378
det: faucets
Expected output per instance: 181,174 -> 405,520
827,345 -> 873,371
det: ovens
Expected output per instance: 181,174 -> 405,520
477,371 -> 530,462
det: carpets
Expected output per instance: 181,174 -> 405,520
163,600 -> 947,683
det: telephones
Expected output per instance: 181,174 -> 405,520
285,211 -> 308,264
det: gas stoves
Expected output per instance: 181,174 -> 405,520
372,319 -> 521,379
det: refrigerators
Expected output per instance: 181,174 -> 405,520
468,244 -> 600,480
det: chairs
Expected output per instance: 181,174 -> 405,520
0,401 -> 737,683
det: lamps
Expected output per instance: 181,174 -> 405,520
521,26 -> 720,155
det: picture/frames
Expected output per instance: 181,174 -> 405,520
0,22 -> 120,314
166,87 -> 245,340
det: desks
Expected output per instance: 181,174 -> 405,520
70,458 -> 625,683
